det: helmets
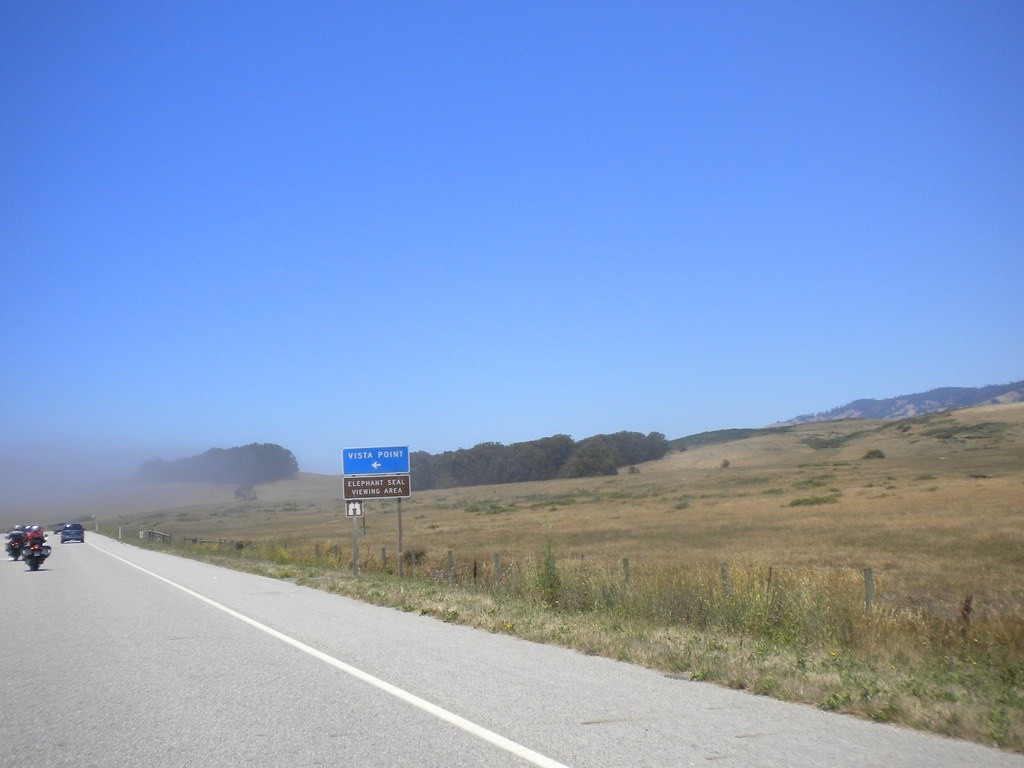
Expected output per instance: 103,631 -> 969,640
15,525 -> 19,530
26,526 -> 32,531
32,526 -> 40,532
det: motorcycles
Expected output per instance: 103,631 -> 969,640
23,534 -> 49,570
5,535 -> 27,561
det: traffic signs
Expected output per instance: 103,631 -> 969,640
343,444 -> 410,475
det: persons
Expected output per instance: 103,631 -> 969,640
22,525 -> 47,561
5,525 -> 26,552
20,525 -> 43,543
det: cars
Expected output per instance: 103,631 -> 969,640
54,522 -> 85,544
